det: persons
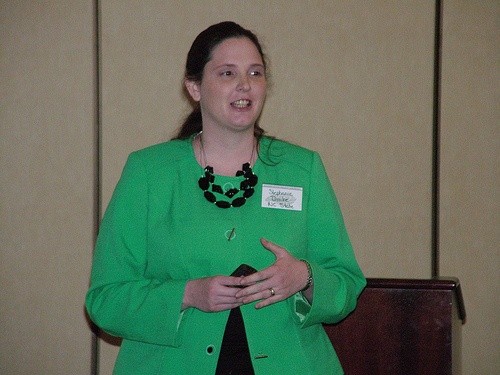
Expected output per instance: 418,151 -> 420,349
84,21 -> 367,375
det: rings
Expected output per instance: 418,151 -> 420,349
269,288 -> 275,295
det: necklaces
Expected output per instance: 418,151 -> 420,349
198,132 -> 258,208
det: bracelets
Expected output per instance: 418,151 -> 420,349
300,260 -> 313,291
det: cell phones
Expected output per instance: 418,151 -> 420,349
225,264 -> 258,288
